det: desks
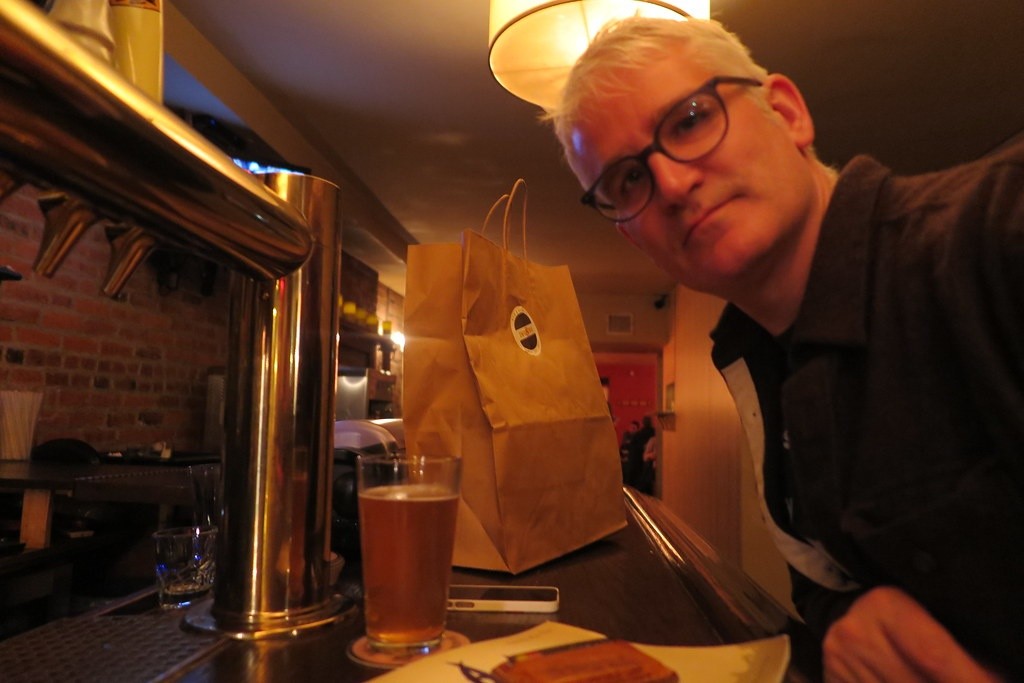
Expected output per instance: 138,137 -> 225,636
175,486 -> 823,683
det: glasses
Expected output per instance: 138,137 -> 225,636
580,75 -> 763,223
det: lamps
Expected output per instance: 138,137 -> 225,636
487,0 -> 710,108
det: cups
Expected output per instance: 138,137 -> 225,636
357,453 -> 460,651
154,525 -> 217,610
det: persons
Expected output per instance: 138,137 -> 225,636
540,17 -> 1024,682
620,416 -> 656,495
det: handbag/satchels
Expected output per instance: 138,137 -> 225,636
402,180 -> 629,574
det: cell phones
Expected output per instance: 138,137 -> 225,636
446,585 -> 559,613
